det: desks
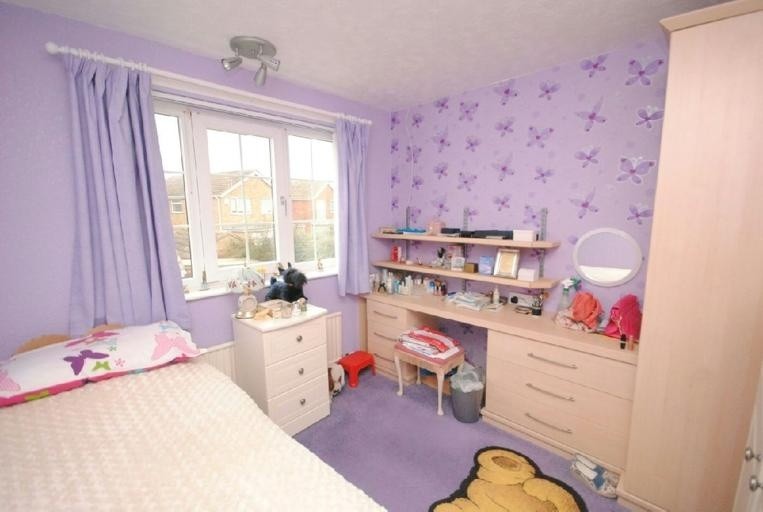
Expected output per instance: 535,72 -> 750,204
393,343 -> 464,416
360,233 -> 639,482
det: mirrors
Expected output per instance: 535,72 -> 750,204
572,226 -> 643,290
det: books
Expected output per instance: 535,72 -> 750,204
446,292 -> 503,314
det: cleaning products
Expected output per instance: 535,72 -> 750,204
558,278 -> 581,314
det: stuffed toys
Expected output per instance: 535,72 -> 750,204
263,262 -> 307,303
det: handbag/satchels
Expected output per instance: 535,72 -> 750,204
569,293 -> 603,328
605,294 -> 641,342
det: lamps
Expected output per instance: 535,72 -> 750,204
220,35 -> 282,87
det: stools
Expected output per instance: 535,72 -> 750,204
338,349 -> 376,387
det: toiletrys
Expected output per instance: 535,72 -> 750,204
492,287 -> 500,304
372,268 -> 445,298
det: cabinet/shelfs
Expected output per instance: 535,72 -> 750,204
231,301 -> 332,440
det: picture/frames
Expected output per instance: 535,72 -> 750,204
492,248 -> 520,279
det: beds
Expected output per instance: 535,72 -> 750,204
0,319 -> 389,512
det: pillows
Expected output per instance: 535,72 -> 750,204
0,321 -> 199,404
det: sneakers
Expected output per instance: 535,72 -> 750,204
570,453 -> 619,499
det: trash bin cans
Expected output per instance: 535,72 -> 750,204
450,367 -> 485,423
